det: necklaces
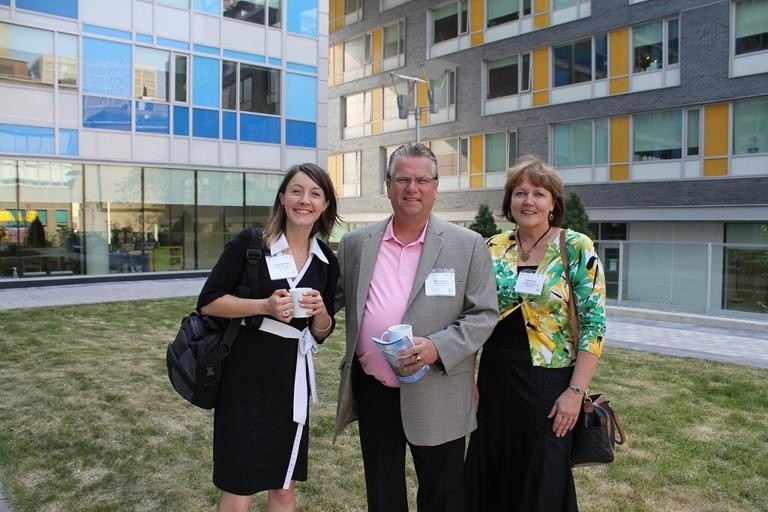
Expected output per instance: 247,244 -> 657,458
514,227 -> 551,261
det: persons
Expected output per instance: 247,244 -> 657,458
197,163 -> 340,512
332,141 -> 501,512
463,156 -> 607,512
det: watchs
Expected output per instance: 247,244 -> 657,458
569,386 -> 589,394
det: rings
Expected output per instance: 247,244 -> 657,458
282,310 -> 289,317
414,355 -> 421,362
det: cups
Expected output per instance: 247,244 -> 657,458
380,324 -> 414,342
283,288 -> 313,318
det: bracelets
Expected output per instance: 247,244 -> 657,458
312,315 -> 332,332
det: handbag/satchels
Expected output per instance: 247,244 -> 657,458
568,388 -> 625,466
166,312 -> 222,410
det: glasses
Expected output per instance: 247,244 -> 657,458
388,175 -> 437,186
